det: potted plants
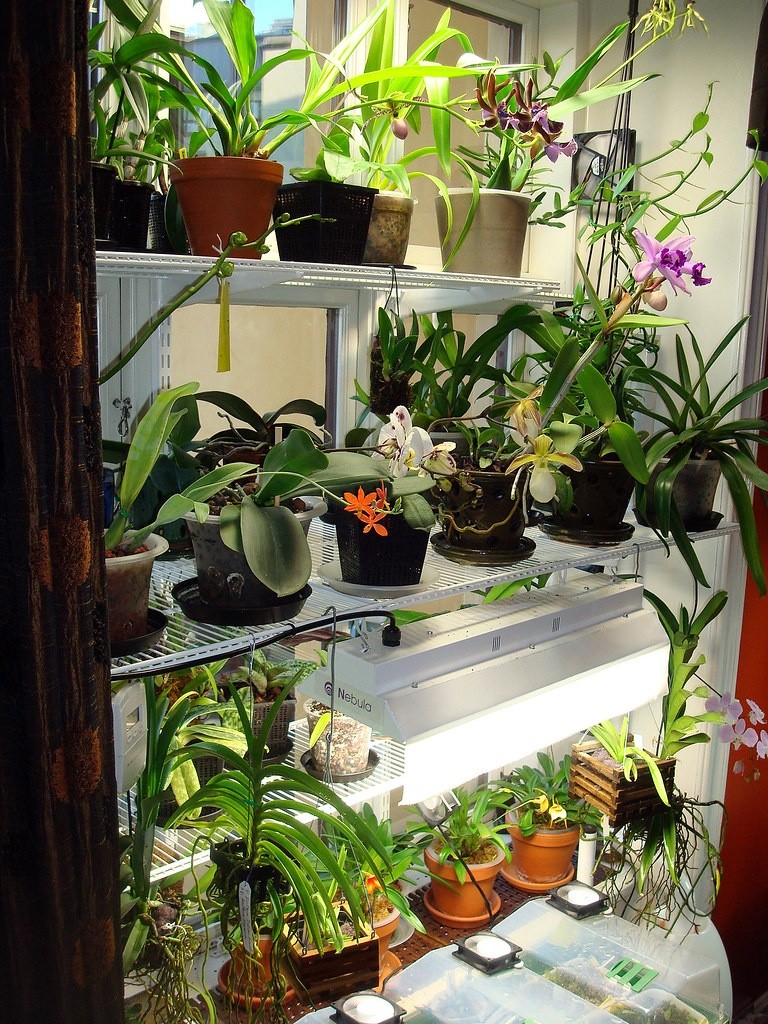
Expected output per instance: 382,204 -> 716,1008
89,0 -> 768,1024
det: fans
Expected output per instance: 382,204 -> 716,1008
568,129 -> 637,205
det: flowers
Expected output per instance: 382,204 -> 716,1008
409,782 -> 566,879
100,368 -> 406,547
484,0 -> 713,197
342,405 -> 462,531
307,801 -> 456,935
497,230 -> 706,433
437,387 -> 588,517
120,3 -> 485,173
428,462 -> 540,568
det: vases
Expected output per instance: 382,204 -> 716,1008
375,907 -> 400,974
107,528 -> 170,656
318,515 -> 441,593
163,157 -> 284,257
485,957 -> 629,1023
436,989 -> 489,1024
422,839 -> 508,929
570,987 -> 709,1024
